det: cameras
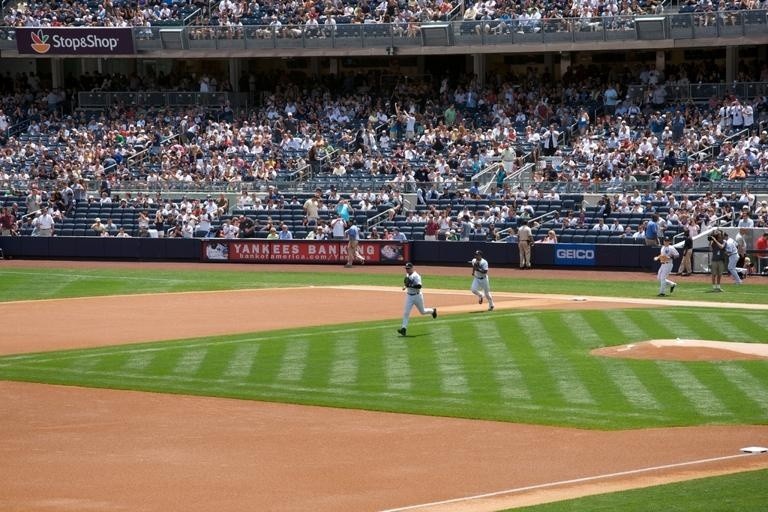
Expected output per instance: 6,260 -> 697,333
343,200 -> 348,204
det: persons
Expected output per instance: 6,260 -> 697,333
0,58 -> 767,271
471,250 -> 495,311
1,1 -> 767,41
397,263 -> 437,336
654,227 -> 767,296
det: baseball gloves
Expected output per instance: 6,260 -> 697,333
404,277 -> 411,286
474,262 -> 481,271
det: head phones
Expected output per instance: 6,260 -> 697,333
719,230 -> 724,237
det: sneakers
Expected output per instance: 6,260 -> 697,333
480,298 -> 483,304
744,269 -> 747,279
397,328 -> 405,336
675,273 -> 692,276
712,287 -> 723,292
658,283 -> 676,297
488,306 -> 495,311
432,308 -> 436,319
520,266 -> 530,269
346,258 -> 365,266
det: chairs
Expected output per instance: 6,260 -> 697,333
0,161 -> 768,244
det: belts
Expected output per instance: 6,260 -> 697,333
407,293 -> 418,296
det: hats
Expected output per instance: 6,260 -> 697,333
663,236 -> 669,240
405,263 -> 413,268
474,250 -> 484,258
